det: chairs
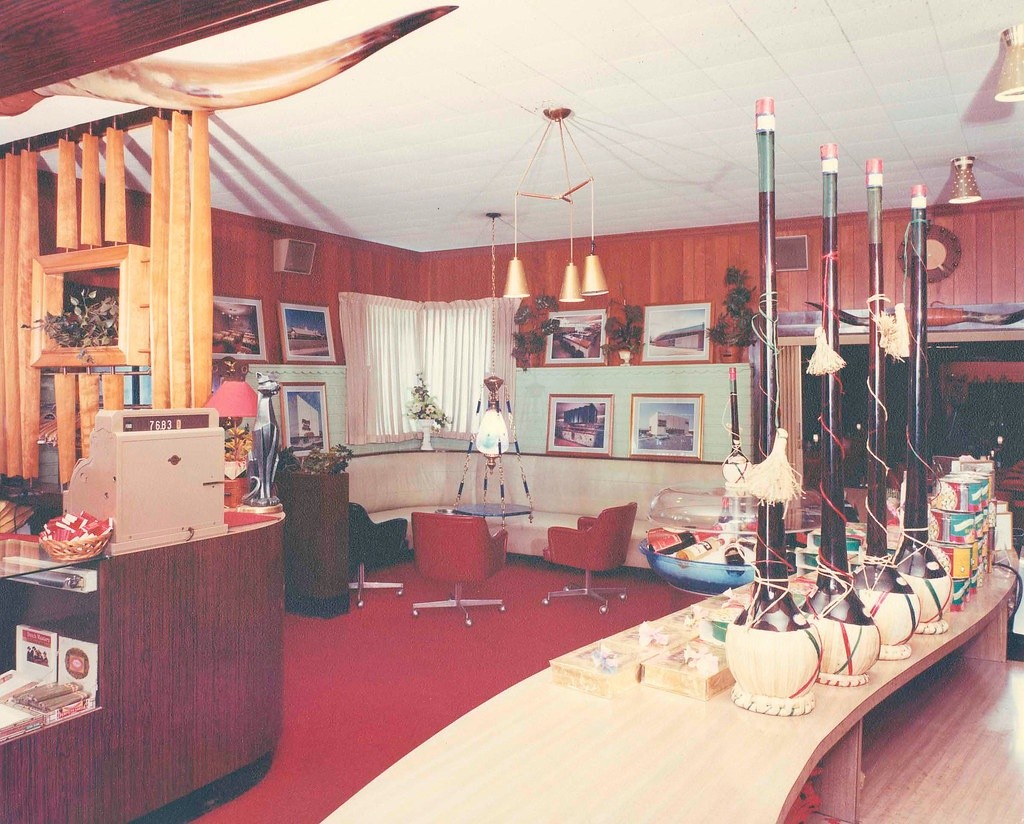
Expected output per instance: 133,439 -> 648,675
347,503 -> 410,606
541,502 -> 638,614
410,511 -> 508,625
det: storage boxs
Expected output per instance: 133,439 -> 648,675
548,580 -> 818,702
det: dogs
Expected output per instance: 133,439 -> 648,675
241,371 -> 282,507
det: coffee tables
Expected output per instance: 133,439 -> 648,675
452,503 -> 533,518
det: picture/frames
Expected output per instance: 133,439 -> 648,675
637,299 -> 714,364
627,393 -> 705,462
276,300 -> 336,364
212,294 -> 269,362
545,393 -> 615,458
278,381 -> 331,461
540,306 -> 609,366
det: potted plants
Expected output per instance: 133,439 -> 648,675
21,285 -> 119,366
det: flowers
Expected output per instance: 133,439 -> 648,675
406,370 -> 453,434
224,422 -> 253,474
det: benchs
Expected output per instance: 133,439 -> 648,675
344,451 -> 774,568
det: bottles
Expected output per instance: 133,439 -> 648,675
646,496 -> 756,577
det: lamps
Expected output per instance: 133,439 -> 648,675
208,378 -> 259,425
502,108 -> 610,302
2,556 -> 98,593
721,367 -> 752,483
454,212 -> 536,529
946,155 -> 982,205
994,23 -> 1024,102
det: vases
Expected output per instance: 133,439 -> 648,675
224,477 -> 249,507
418,418 -> 436,450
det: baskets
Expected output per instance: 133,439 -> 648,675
38,525 -> 113,561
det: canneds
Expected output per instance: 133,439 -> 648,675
926,471 -> 994,613
